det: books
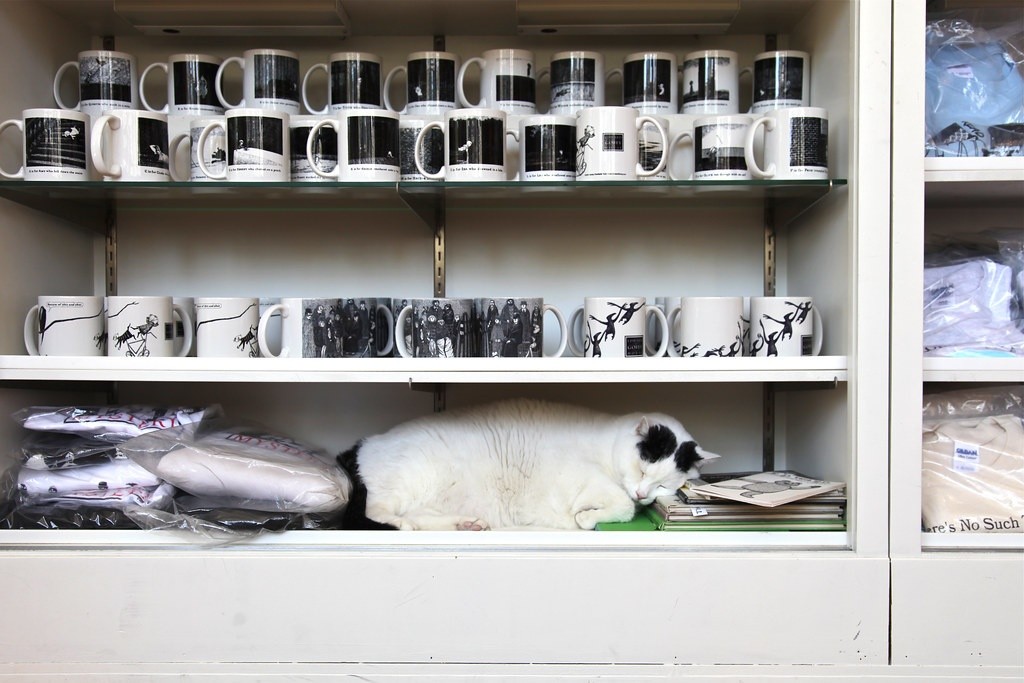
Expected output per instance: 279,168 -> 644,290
595,469 -> 847,533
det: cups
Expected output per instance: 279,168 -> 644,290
170,297 -> 199,356
25,296 -> 108,359
1,44 -> 830,191
565,296 -> 824,358
254,297 -> 285,358
194,297 -> 261,359
105,293 -> 193,357
257,299 -> 568,365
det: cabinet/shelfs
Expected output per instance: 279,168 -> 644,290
888,2 -> 1024,680
0,2 -> 888,680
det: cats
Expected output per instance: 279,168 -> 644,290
327,396 -> 723,532
